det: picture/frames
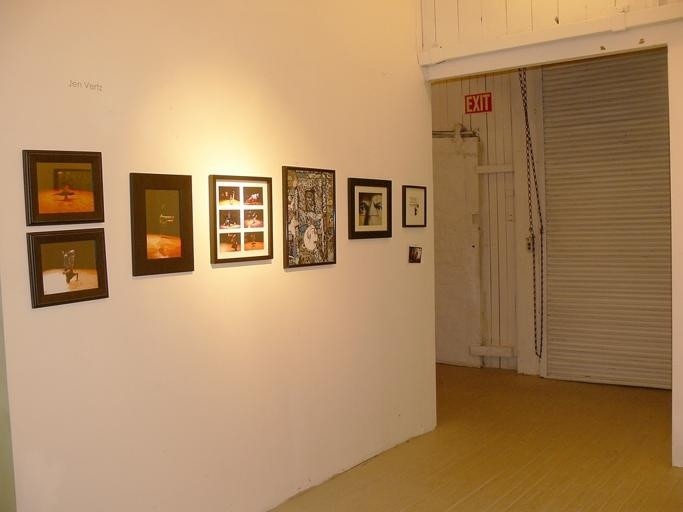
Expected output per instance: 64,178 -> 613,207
402,185 -> 426,227
282,166 -> 336,268
409,247 -> 422,263
348,178 -> 392,239
209,175 -> 273,264
26,228 -> 108,308
22,150 -> 104,226
130,173 -> 194,276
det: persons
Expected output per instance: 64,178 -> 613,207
357,193 -> 382,227
218,188 -> 262,251
62,249 -> 79,284
158,208 -> 176,226
49,186 -> 83,201
